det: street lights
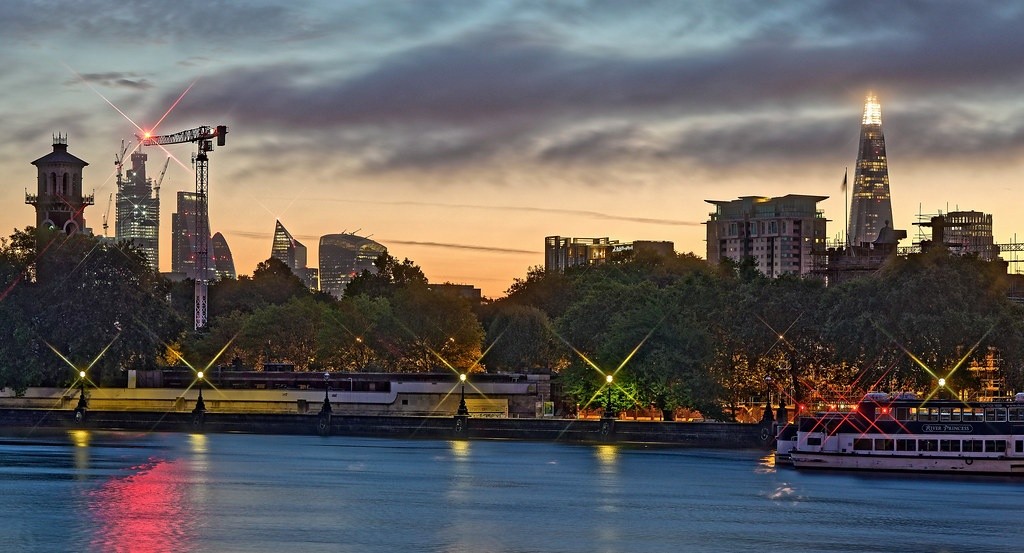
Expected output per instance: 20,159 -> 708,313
192,371 -> 208,412
319,371 -> 335,414
456,373 -> 471,417
604,374 -> 615,418
73,371 -> 91,410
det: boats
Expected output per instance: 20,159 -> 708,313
774,392 -> 1024,476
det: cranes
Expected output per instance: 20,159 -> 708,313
140,124 -> 229,328
